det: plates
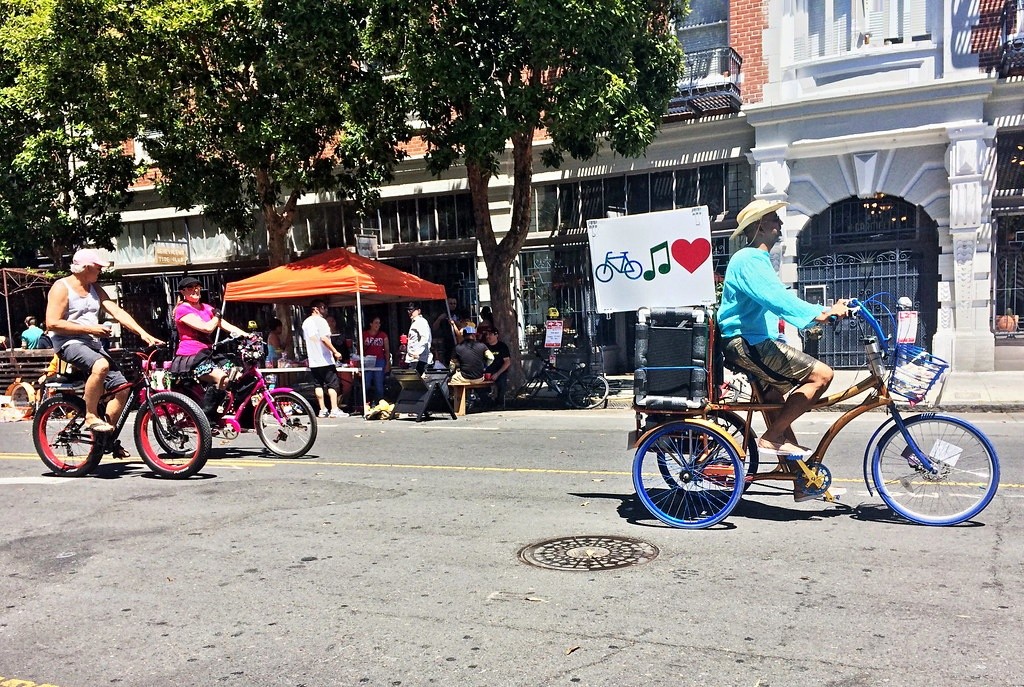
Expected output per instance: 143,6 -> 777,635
331,333 -> 340,337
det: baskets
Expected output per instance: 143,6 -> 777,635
887,344 -> 950,401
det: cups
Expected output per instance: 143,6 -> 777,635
346,339 -> 353,347
399,335 -> 408,344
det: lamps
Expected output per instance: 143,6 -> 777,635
864,33 -> 870,45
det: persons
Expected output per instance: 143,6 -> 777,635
267,318 -> 293,415
356,315 -> 390,408
170,275 -> 263,437
404,301 -> 434,417
716,199 -> 855,502
302,299 -> 349,417
324,315 -> 352,408
429,292 -> 511,412
45,249 -> 169,458
0,316 -> 119,350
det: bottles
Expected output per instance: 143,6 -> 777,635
142,361 -> 172,391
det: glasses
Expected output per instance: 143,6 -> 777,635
184,285 -> 201,290
317,305 -> 326,309
449,302 -> 457,305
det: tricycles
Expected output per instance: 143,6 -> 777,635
630,295 -> 1001,527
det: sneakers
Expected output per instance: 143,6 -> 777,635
330,409 -> 349,418
319,410 -> 328,417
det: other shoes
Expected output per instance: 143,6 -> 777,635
758,438 -> 812,457
241,421 -> 264,430
794,485 -> 846,502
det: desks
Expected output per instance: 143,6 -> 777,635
255,367 -> 382,416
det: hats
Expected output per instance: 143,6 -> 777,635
729,198 -> 791,242
462,326 -> 476,334
178,277 -> 202,288
404,301 -> 421,311
484,327 -> 499,334
73,250 -> 111,268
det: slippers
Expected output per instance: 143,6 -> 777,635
107,444 -> 131,458
85,419 -> 113,432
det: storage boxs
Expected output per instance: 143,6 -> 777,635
351,354 -> 377,368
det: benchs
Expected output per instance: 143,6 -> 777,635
448,378 -> 511,418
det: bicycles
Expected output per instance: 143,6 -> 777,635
510,349 -> 610,410
32,342 -> 213,479
142,333 -> 319,459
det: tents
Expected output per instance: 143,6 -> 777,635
214,245 -> 457,418
